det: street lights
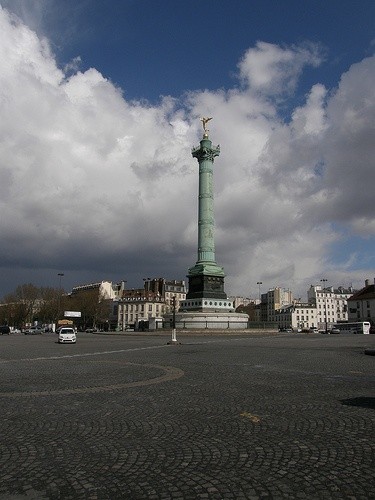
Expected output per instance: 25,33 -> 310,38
320,279 -> 328,335
257,281 -> 262,328
57,273 -> 64,320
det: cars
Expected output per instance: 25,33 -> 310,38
85,326 -> 104,333
24,326 -> 44,335
56,324 -> 77,334
57,327 -> 77,344
278,326 -> 340,335
0,325 -> 10,336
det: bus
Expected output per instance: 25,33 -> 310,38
329,321 -> 371,335
